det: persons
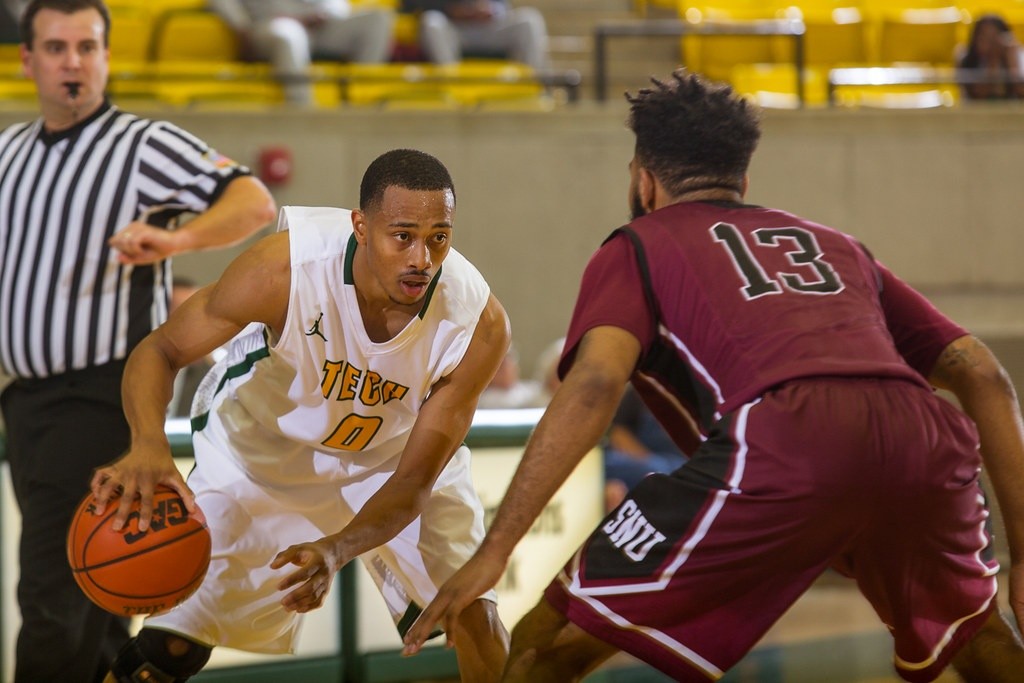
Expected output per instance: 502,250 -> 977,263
90,148 -> 513,682
394,1 -> 547,70
400,81 -> 1024,683
955,14 -> 1024,107
209,0 -> 390,101
0,0 -> 278,682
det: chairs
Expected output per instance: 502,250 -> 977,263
0,0 -> 1024,110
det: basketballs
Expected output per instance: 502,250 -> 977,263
67,482 -> 212,618
